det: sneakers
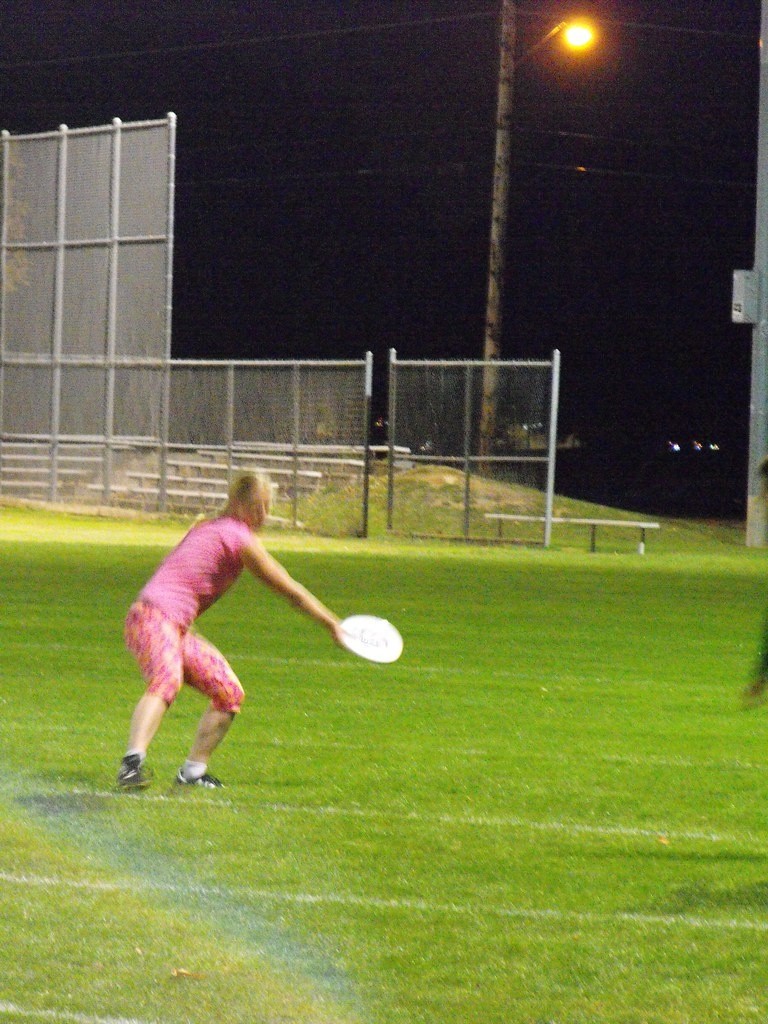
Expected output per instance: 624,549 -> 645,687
174,769 -> 225,790
116,755 -> 144,785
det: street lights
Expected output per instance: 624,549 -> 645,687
475,16 -> 594,478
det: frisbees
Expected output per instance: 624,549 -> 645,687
341,614 -> 403,664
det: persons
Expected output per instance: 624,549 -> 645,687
113,473 -> 342,788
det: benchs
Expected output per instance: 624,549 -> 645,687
0,433 -> 411,506
483,511 -> 658,555
